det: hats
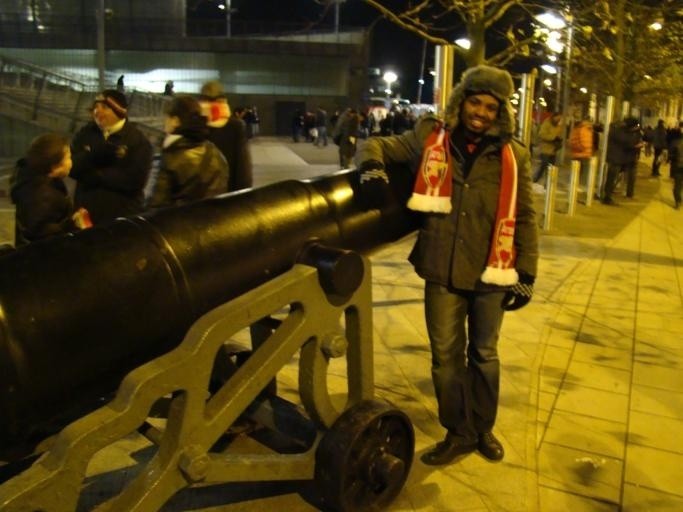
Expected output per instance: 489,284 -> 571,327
444,66 -> 515,138
623,116 -> 639,127
93,89 -> 126,119
200,81 -> 222,98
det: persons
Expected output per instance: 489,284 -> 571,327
8,137 -> 92,248
140,98 -> 230,212
117,74 -> 124,89
70,90 -> 154,230
294,101 -> 417,166
232,102 -> 259,131
354,65 -> 538,466
164,81 -> 174,95
534,109 -> 683,210
200,80 -> 253,192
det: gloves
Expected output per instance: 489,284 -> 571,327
500,273 -> 533,311
552,137 -> 563,146
357,161 -> 389,210
73,208 -> 92,227
348,137 -> 356,146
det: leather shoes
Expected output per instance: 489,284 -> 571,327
421,437 -> 478,465
476,432 -> 504,459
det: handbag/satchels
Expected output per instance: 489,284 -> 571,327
331,132 -> 343,146
